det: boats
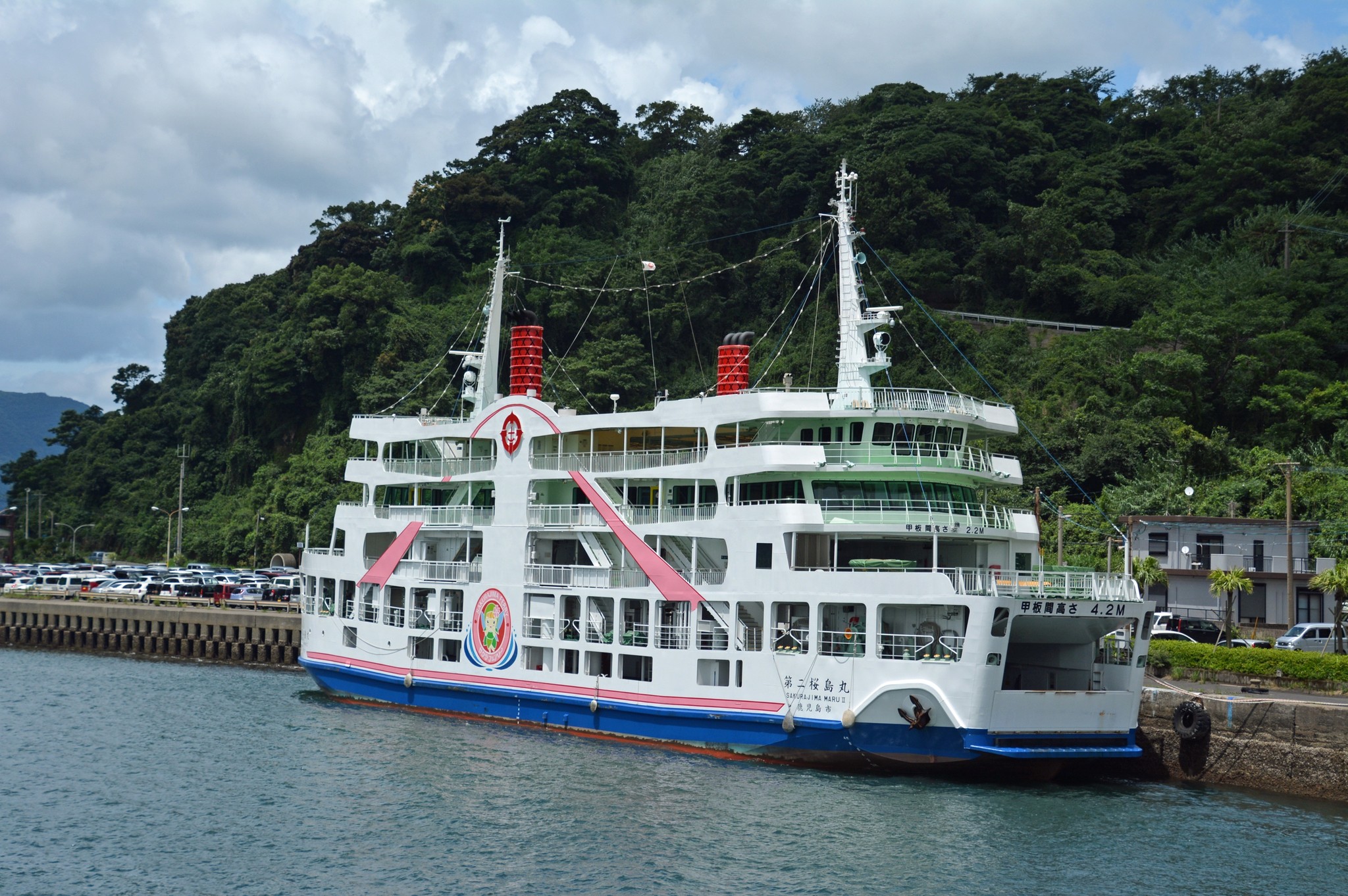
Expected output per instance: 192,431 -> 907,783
298,158 -> 1156,777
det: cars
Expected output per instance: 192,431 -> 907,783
1216,640 -> 1271,651
1150,629 -> 1199,645
0,562 -> 301,612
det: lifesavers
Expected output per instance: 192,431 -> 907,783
1171,701 -> 1212,742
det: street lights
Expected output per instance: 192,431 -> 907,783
253,514 -> 265,567
151,506 -> 190,568
55,522 -> 95,557
1057,505 -> 1072,567
25,487 -> 30,539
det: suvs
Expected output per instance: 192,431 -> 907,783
1167,617 -> 1237,643
1130,612 -> 1182,630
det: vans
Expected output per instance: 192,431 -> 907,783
88,551 -> 105,563
1274,622 -> 1348,656
102,552 -> 118,566
